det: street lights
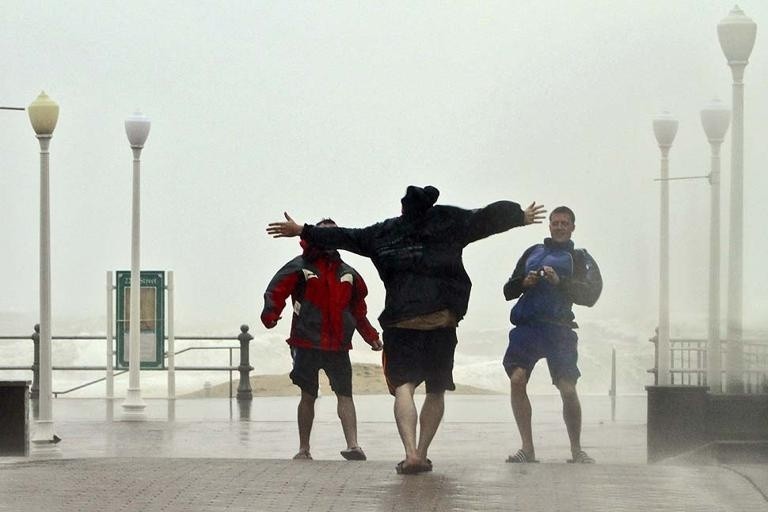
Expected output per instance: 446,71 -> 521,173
653,104 -> 681,387
26,86 -> 63,443
698,2 -> 758,394
120,106 -> 152,422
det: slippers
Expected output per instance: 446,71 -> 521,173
340,446 -> 365,459
508,451 -> 540,464
396,460 -> 433,475
567,452 -> 593,463
293,450 -> 312,460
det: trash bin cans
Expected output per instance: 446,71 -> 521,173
0,380 -> 32,456
645,386 -> 709,464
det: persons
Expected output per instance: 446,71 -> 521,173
501,206 -> 604,465
260,217 -> 382,462
265,184 -> 547,472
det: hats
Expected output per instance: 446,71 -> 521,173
402,187 -> 438,213
